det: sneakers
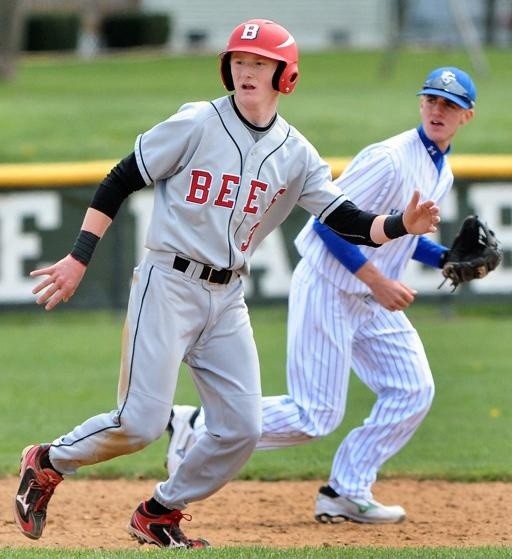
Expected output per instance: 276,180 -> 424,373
126,500 -> 210,551
13,443 -> 65,540
165,404 -> 201,477
314,486 -> 406,523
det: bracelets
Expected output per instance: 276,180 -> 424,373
70,228 -> 102,263
381,209 -> 409,243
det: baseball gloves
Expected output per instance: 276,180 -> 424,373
438,215 -> 502,293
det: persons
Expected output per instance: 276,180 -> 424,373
12,15 -> 441,552
163,65 -> 503,529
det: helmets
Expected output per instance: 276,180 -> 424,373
216,18 -> 300,96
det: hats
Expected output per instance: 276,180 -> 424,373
416,67 -> 477,109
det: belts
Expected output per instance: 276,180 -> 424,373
173,255 -> 233,286
